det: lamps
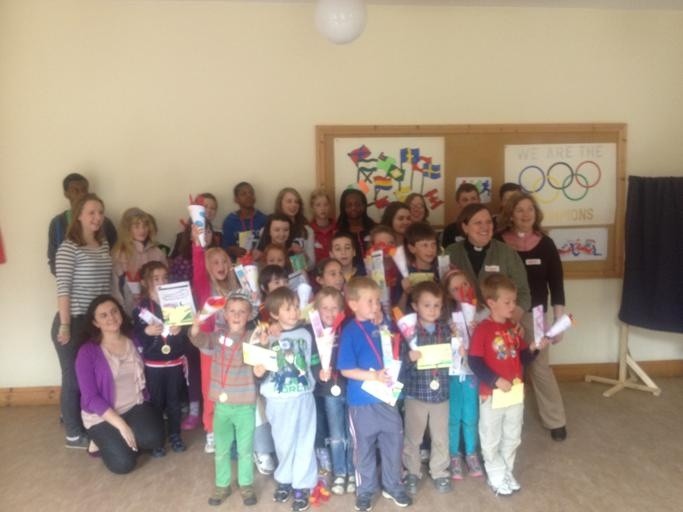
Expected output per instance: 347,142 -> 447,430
312,0 -> 373,47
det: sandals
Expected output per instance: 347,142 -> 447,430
86,445 -> 101,458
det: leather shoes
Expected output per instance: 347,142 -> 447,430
550,427 -> 567,441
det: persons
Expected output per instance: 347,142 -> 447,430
47,174 -> 572,511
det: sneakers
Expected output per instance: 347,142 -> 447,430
381,471 -> 418,507
434,474 -> 453,494
204,431 -> 215,454
181,415 -> 203,431
236,480 -> 257,506
310,473 -> 357,505
289,488 -> 313,512
167,434 -> 186,452
208,484 -> 234,506
486,469 -> 521,496
354,492 -> 375,511
272,482 -> 291,503
64,434 -> 88,449
252,451 -> 277,476
451,453 -> 483,480
149,444 -> 167,458
